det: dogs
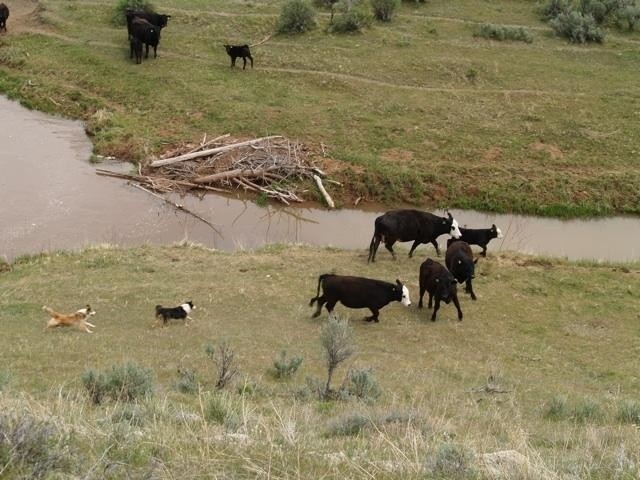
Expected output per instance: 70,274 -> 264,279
152,300 -> 196,330
42,303 -> 96,335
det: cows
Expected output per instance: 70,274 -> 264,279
451,223 -> 504,258
445,240 -> 477,301
0,3 -> 9,33
366,208 -> 464,264
125,9 -> 171,64
418,257 -> 463,323
223,44 -> 253,70
308,273 -> 411,324
